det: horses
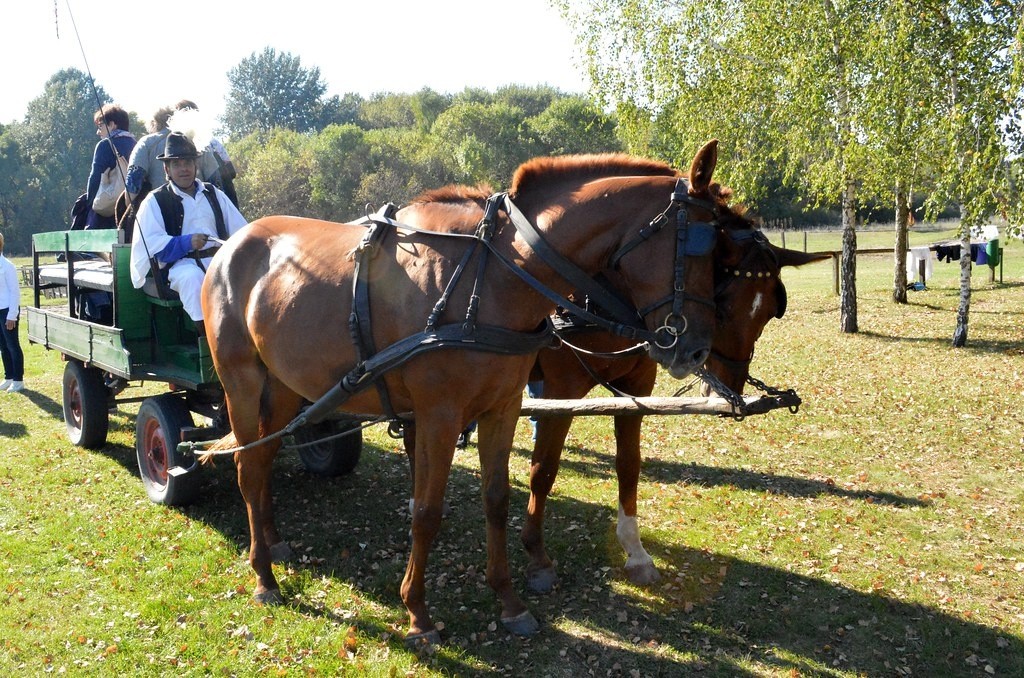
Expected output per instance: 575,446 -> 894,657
199,139 -> 837,647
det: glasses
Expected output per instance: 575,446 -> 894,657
96,122 -> 108,129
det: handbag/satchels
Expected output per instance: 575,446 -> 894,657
92,138 -> 128,218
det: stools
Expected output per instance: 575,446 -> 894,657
144,294 -> 187,365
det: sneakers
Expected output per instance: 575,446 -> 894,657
7,381 -> 24,392
0,379 -> 12,390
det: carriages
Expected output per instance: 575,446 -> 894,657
23,137 -> 843,657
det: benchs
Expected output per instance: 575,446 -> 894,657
42,260 -> 114,320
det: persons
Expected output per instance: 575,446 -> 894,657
130,131 -> 248,337
124,106 -> 223,222
0,233 -> 25,392
71,104 -> 137,231
175,100 -> 239,211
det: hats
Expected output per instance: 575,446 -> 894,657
156,130 -> 204,161
